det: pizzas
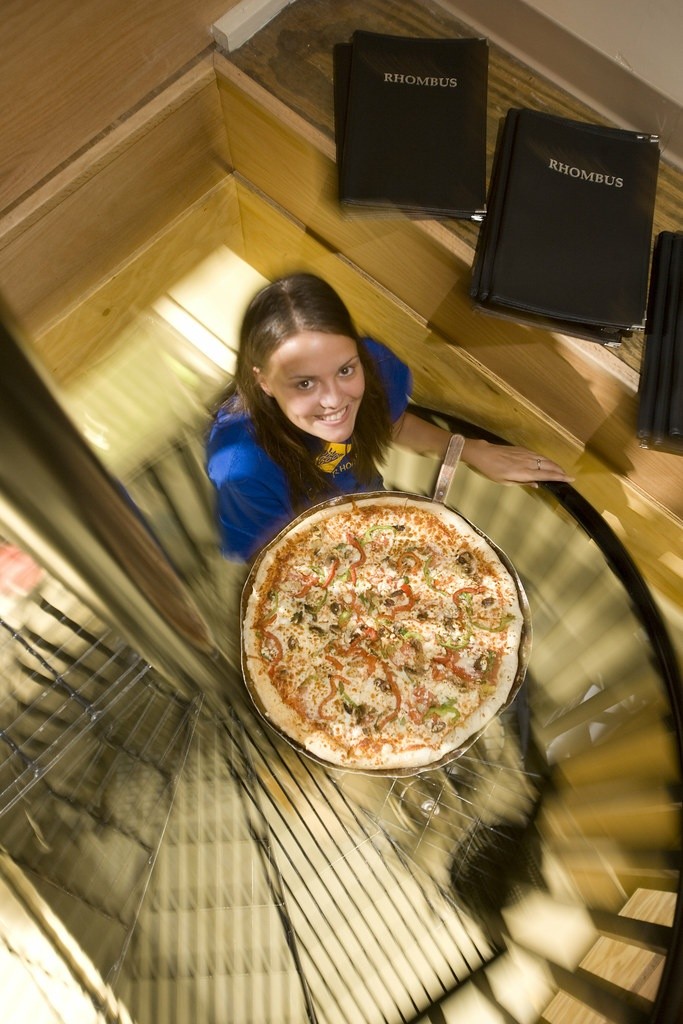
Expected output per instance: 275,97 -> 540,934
242,496 -> 524,770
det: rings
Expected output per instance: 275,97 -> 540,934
537,459 -> 541,470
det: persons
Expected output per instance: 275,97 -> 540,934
202,273 -> 577,569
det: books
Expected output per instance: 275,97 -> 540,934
329,29 -> 683,446
